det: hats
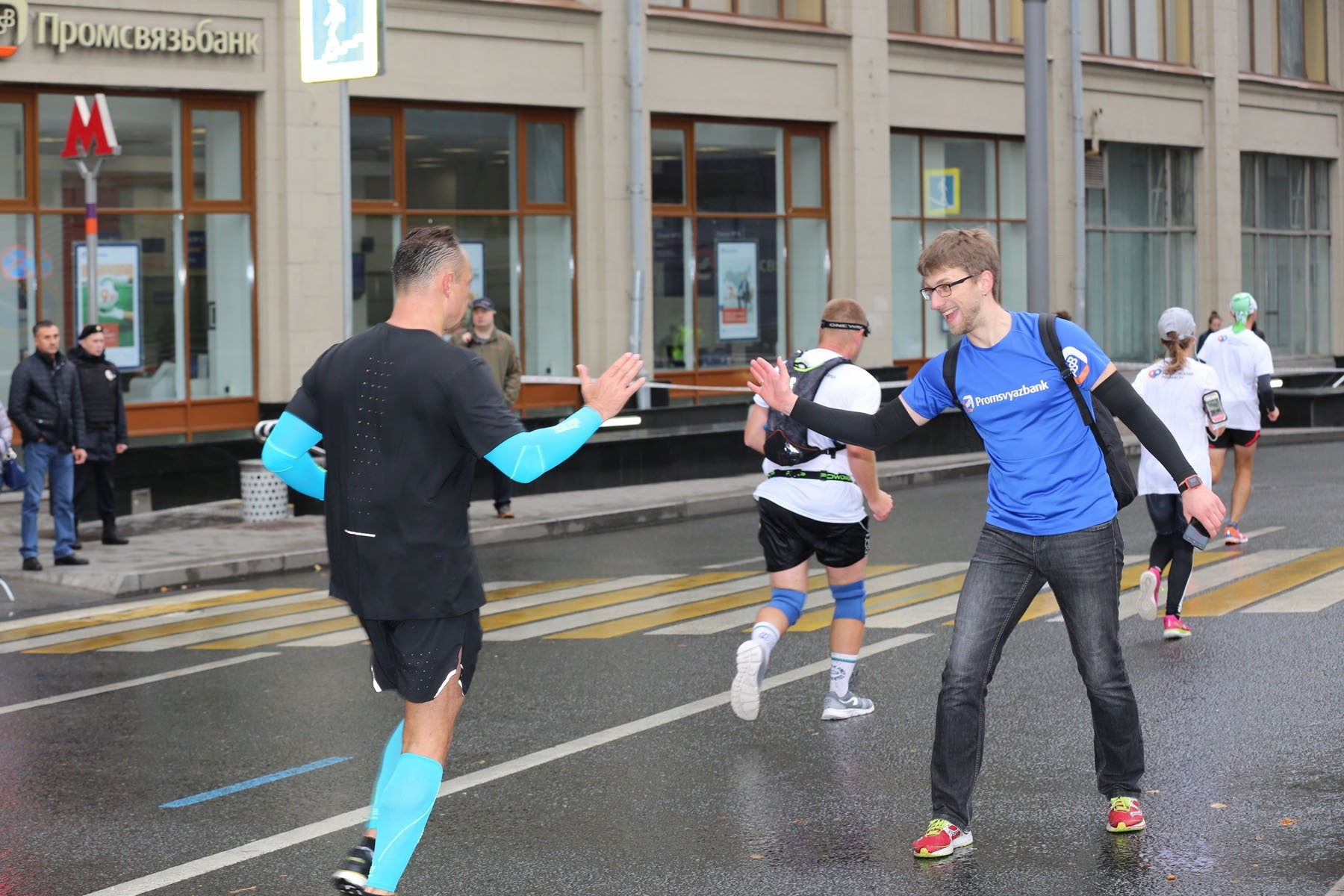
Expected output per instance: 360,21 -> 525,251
473,296 -> 494,310
1159,307 -> 1195,342
78,324 -> 103,339
1229,292 -> 1258,333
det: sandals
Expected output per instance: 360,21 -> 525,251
1224,525 -> 1249,543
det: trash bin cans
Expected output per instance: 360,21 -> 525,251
237,459 -> 289,523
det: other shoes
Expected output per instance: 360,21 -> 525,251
55,556 -> 88,565
498,504 -> 514,518
70,543 -> 82,549
103,534 -> 129,544
330,846 -> 375,896
23,557 -> 42,571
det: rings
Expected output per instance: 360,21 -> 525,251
760,380 -> 765,384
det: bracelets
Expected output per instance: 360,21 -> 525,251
1178,474 -> 1203,495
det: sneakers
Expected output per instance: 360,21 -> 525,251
911,819 -> 975,859
729,643 -> 767,720
1138,567 -> 1161,622
1106,794 -> 1146,833
820,693 -> 874,720
1163,615 -> 1191,638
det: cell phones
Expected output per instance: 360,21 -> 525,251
1182,516 -> 1210,550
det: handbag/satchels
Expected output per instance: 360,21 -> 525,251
3,438 -> 29,490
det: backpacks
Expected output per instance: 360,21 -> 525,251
944,314 -> 1137,515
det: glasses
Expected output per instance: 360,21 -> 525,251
920,272 -> 982,302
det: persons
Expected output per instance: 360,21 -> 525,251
745,226 -> 1225,860
67,328 -> 129,549
1197,292 -> 1280,545
1196,310 -> 1223,356
1251,320 -> 1266,341
731,299 -> 880,721
0,401 -> 13,496
8,321 -> 88,571
1130,307 -> 1226,638
456,297 -> 522,520
261,225 -> 645,896
